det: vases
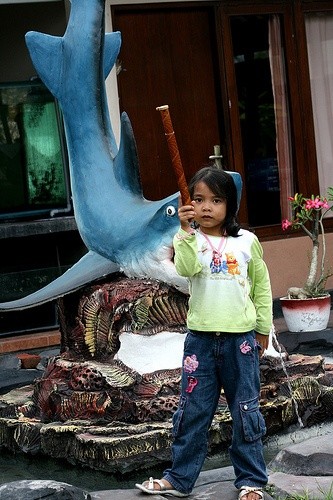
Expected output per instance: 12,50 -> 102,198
280,294 -> 331,333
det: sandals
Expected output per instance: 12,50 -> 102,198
135,476 -> 190,498
237,485 -> 263,500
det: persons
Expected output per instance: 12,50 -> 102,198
135,167 -> 273,500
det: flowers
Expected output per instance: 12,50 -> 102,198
282,186 -> 333,299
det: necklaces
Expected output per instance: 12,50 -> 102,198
199,227 -> 228,274
